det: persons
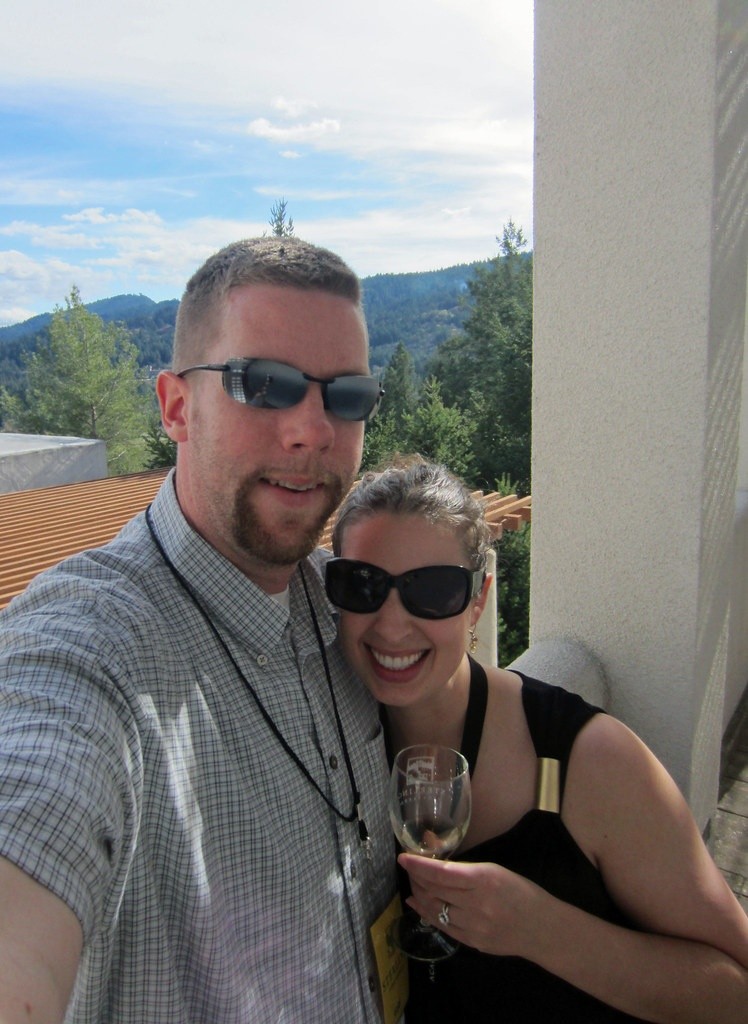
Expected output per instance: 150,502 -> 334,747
332,458 -> 748,1024
0,237 -> 404,1024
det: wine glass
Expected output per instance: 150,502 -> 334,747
385,745 -> 473,963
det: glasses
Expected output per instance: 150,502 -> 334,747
173,357 -> 390,422
324,555 -> 484,620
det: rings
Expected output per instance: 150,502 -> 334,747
438,904 -> 451,925
